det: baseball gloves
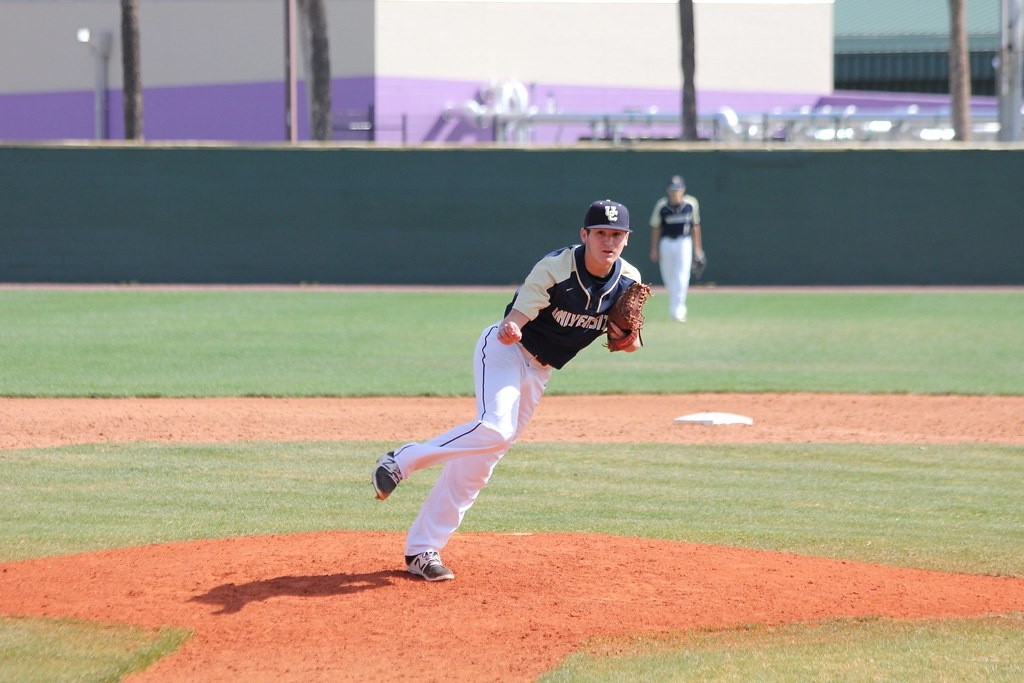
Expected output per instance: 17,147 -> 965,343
607,282 -> 651,352
692,255 -> 707,277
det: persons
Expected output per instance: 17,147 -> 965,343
370,198 -> 650,582
649,173 -> 706,323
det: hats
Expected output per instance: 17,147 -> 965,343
666,175 -> 684,190
583,199 -> 632,233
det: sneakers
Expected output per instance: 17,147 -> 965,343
405,551 -> 454,582
371,451 -> 403,501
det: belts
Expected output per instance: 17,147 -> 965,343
522,344 -> 547,367
